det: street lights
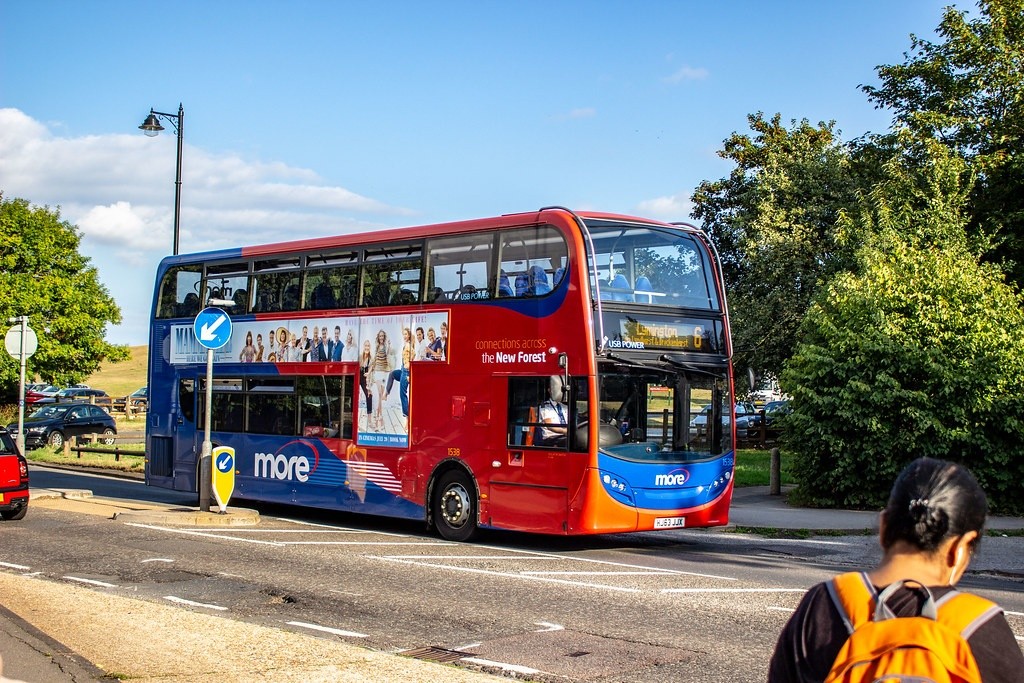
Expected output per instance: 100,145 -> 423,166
136,100 -> 185,256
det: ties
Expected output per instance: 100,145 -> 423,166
557,403 -> 566,424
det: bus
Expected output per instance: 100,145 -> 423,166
144,204 -> 757,542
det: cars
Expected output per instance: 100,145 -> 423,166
691,370 -> 794,438
113,387 -> 146,412
24,382 -> 114,414
6,403 -> 118,452
0,426 -> 30,520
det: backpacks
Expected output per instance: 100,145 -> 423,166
822,571 -> 1004,683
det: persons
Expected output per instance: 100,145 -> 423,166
240,322 -> 448,431
364,330 -> 391,431
539,388 -> 568,447
768,457 -> 1024,683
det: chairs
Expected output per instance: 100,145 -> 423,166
224,405 -> 352,439
78,409 -> 86,417
183,266 -> 657,318
526,406 -> 538,446
